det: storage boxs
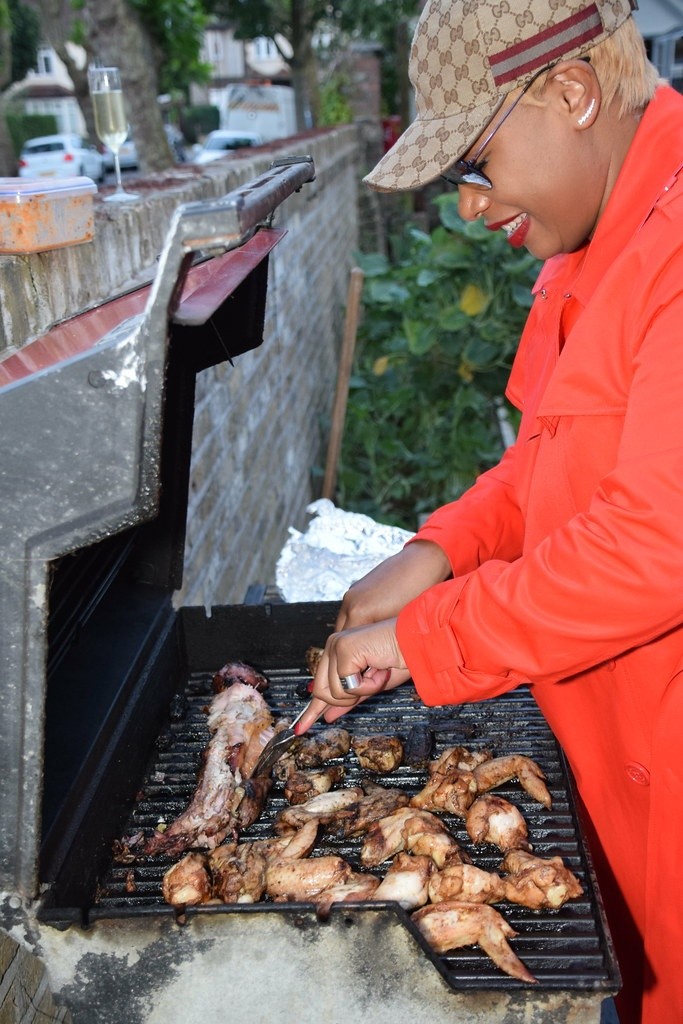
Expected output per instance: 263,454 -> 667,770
0,174 -> 98,254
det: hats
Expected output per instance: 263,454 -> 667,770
362,0 -> 639,192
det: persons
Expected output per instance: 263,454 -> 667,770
294,1 -> 683,1024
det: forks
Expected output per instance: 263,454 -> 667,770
250,665 -> 371,781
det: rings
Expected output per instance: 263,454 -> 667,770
339,671 -> 365,690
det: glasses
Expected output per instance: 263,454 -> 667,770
440,58 -> 593,190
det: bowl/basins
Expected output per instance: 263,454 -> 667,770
0,178 -> 102,255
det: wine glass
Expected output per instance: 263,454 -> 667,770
90,65 -> 141,203
